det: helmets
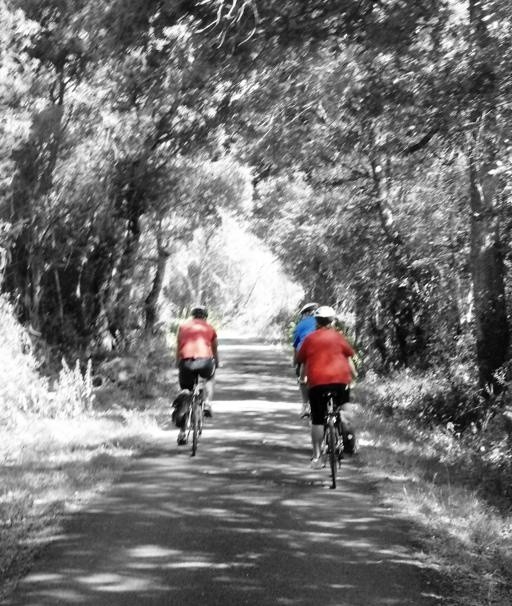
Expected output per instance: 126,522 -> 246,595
191,305 -> 208,317
300,302 -> 319,314
313,305 -> 337,326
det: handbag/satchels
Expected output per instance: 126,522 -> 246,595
339,412 -> 357,455
172,388 -> 193,427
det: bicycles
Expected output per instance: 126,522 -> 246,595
178,365 -> 223,456
323,392 -> 344,488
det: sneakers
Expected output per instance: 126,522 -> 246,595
202,404 -> 213,418
177,433 -> 186,442
300,406 -> 310,419
308,457 -> 324,469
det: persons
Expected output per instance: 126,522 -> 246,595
290,302 -> 319,420
294,305 -> 359,469
173,305 -> 224,446
